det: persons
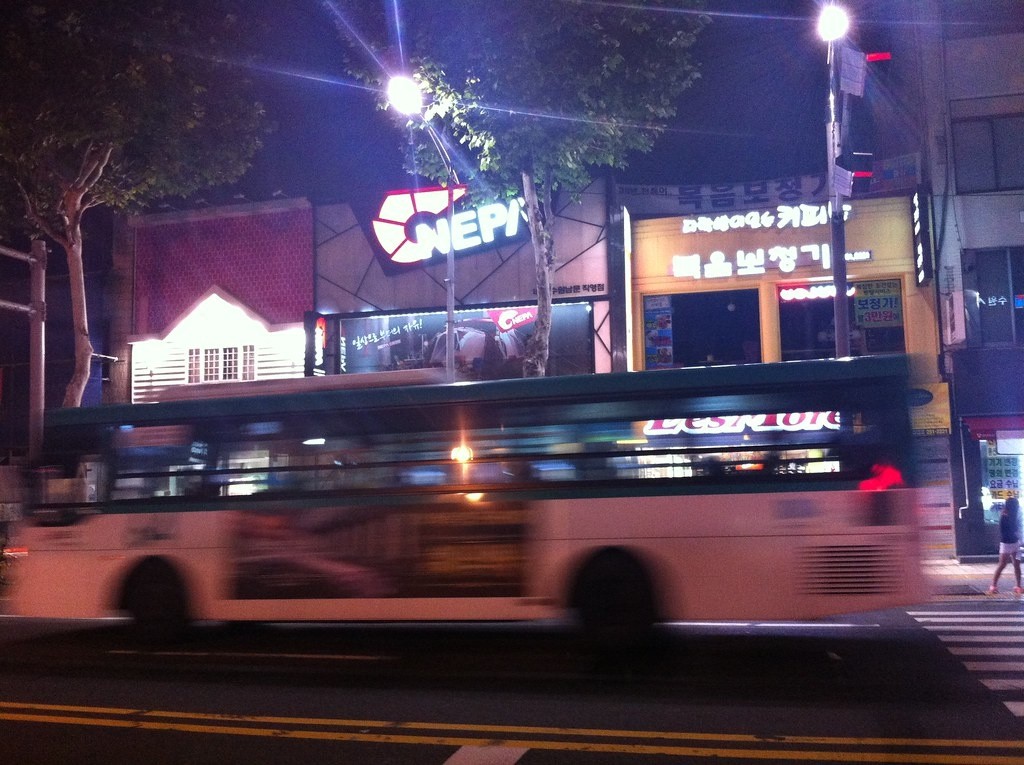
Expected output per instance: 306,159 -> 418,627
989,498 -> 1024,593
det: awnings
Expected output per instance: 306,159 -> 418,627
963,416 -> 1024,441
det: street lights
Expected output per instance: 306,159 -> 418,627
821,11 -> 857,359
387,77 -> 457,383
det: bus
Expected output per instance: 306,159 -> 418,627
2,369 -> 931,657
